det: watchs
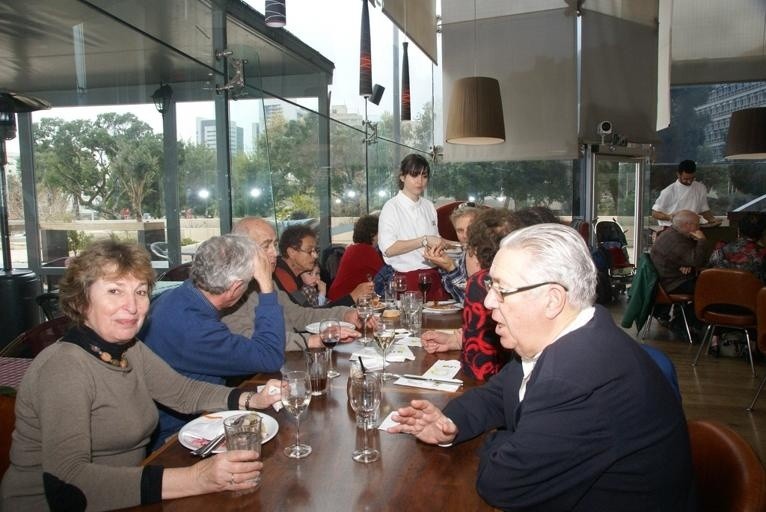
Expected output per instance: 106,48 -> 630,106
421,235 -> 428,246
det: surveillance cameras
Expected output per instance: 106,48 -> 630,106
597,120 -> 613,135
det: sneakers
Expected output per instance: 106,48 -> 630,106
670,318 -> 701,344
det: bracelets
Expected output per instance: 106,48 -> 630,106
244,391 -> 256,411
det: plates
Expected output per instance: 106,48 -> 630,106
176,409 -> 279,454
443,240 -> 464,259
305,322 -> 355,335
423,301 -> 464,312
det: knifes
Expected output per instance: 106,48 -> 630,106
402,375 -> 464,388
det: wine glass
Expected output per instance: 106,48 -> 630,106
357,273 -> 433,344
374,320 -> 394,381
280,370 -> 312,460
350,371 -> 380,464
318,319 -> 341,379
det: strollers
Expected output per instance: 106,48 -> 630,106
592,216 -> 637,300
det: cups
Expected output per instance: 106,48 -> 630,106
224,412 -> 263,495
304,350 -> 329,397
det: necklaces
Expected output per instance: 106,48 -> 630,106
90,345 -> 128,369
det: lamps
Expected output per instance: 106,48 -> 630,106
400,0 -> 411,120
359,0 -> 372,96
724,107 -> 766,159
152,82 -> 172,119
265,0 -> 285,27
445,0 -> 505,145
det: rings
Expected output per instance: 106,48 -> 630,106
369,287 -> 372,290
231,473 -> 234,482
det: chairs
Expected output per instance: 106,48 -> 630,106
151,242 -> 168,259
159,262 -> 191,281
745,288 -> 766,412
596,220 -> 634,305
636,253 -> 693,344
322,246 -> 345,291
691,269 -> 761,379
688,418 -> 766,512
0,316 -> 71,481
36,292 -> 61,319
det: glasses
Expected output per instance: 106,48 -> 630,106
290,244 -> 321,255
482,273 -> 571,304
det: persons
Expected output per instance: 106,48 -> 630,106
134,233 -> 287,458
420,202 -> 484,306
0,238 -> 290,512
214,216 -> 378,352
327,214 -> 383,306
647,210 -> 708,333
384,222 -> 697,511
651,159 -> 722,226
299,260 -> 330,307
701,212 -> 766,360
417,207 -> 527,383
273,223 -> 374,309
377,153 -> 452,304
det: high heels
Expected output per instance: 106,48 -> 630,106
708,344 -> 722,358
